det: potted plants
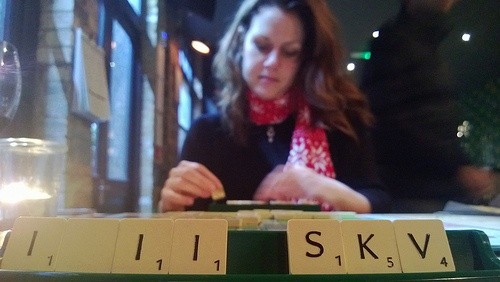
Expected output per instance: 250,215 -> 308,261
454,74 -> 500,207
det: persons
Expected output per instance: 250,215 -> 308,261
357,0 -> 500,213
159,0 -> 392,215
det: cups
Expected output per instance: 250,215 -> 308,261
0,138 -> 62,218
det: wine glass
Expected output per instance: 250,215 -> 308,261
0,39 -> 22,138
